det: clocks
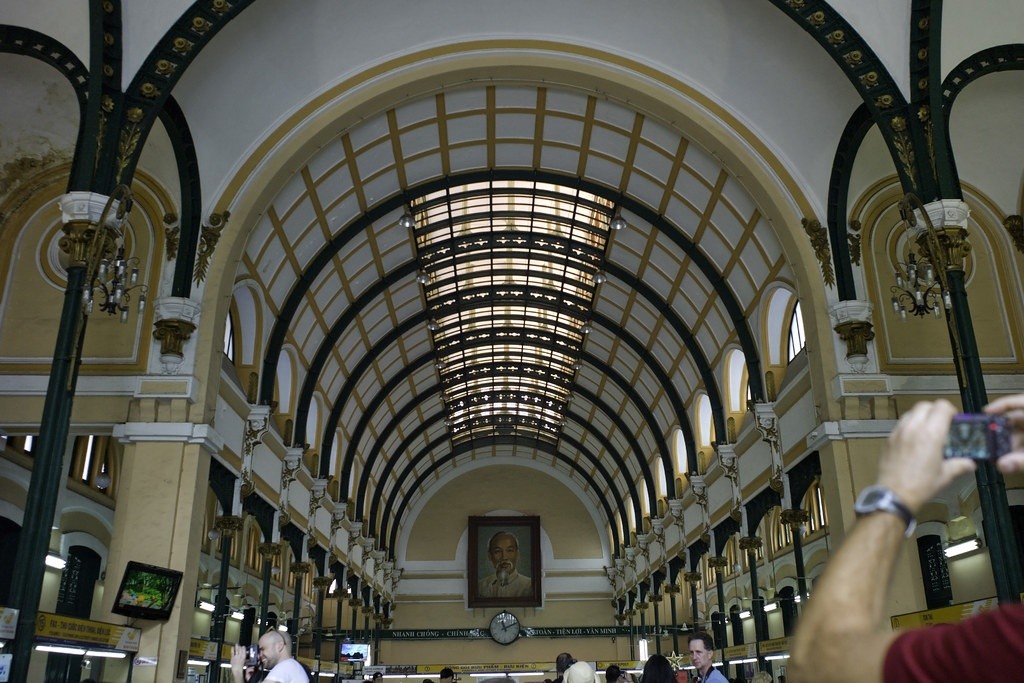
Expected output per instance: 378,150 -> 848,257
489,610 -> 520,646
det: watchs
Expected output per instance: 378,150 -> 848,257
854,485 -> 917,539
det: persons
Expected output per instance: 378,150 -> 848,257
439,668 -> 454,683
606,665 -> 634,683
556,653 -> 594,683
372,672 -> 383,683
687,632 -> 729,683
230,630 -> 309,683
786,393 -> 1024,683
640,654 -> 678,683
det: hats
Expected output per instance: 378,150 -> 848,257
373,672 -> 383,679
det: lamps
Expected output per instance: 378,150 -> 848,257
890,192 -> 966,387
66,183 -> 150,391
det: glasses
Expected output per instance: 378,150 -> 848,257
266,626 -> 286,645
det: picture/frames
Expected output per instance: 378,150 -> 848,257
468,515 -> 542,608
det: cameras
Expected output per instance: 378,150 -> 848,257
246,644 -> 260,666
943,414 -> 1013,460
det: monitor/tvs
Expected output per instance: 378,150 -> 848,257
110,561 -> 183,621
